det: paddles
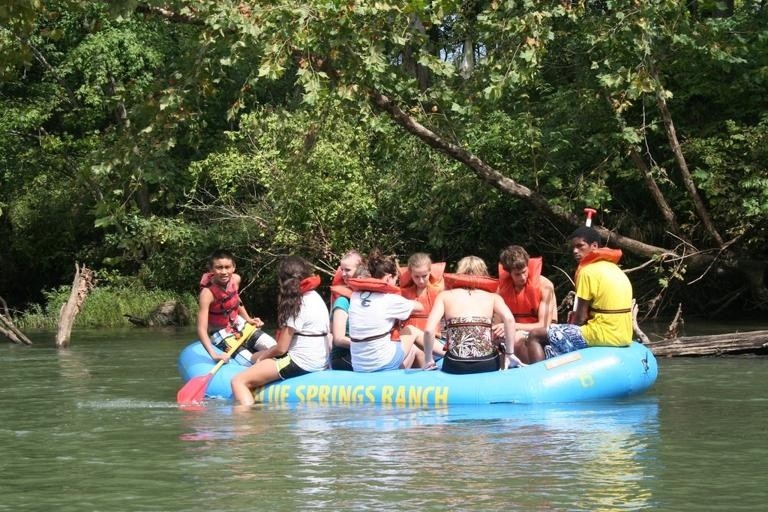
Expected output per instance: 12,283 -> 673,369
176,325 -> 256,405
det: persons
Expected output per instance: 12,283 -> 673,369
229,256 -> 332,409
194,250 -> 278,367
514,226 -> 633,364
491,245 -> 557,338
329,248 -> 530,375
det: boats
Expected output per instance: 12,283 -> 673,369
179,338 -> 658,405
